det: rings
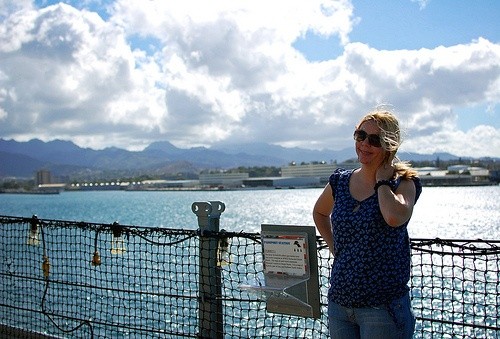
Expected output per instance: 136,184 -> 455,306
385,165 -> 388,168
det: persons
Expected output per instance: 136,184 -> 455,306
313,108 -> 422,339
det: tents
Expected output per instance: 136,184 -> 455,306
448,165 -> 488,175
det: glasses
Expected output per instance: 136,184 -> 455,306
353,129 -> 382,147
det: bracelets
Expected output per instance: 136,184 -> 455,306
374,179 -> 392,189
